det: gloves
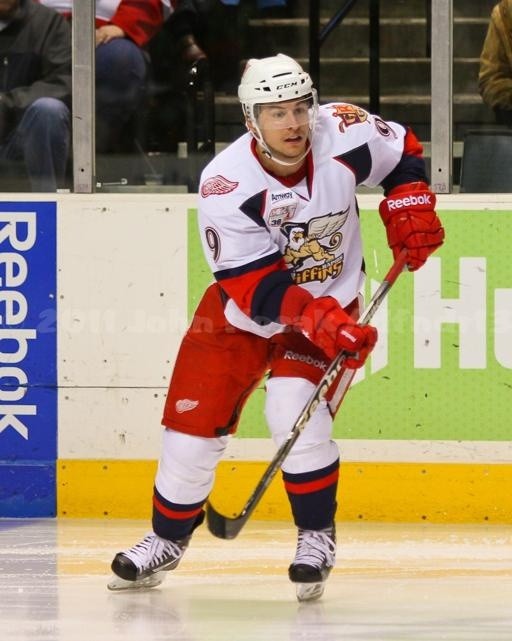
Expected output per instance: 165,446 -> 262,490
300,296 -> 377,368
379,181 -> 445,271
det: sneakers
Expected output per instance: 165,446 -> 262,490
111,534 -> 191,581
288,523 -> 336,582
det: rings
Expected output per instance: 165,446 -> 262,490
100,38 -> 103,41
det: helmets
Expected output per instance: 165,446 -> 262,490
238,53 -> 317,131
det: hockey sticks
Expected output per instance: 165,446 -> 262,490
206,250 -> 406,542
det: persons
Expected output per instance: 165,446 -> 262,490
110,49 -> 443,583
43,0 -> 173,188
477,1 -> 511,127
1,1 -> 73,192
